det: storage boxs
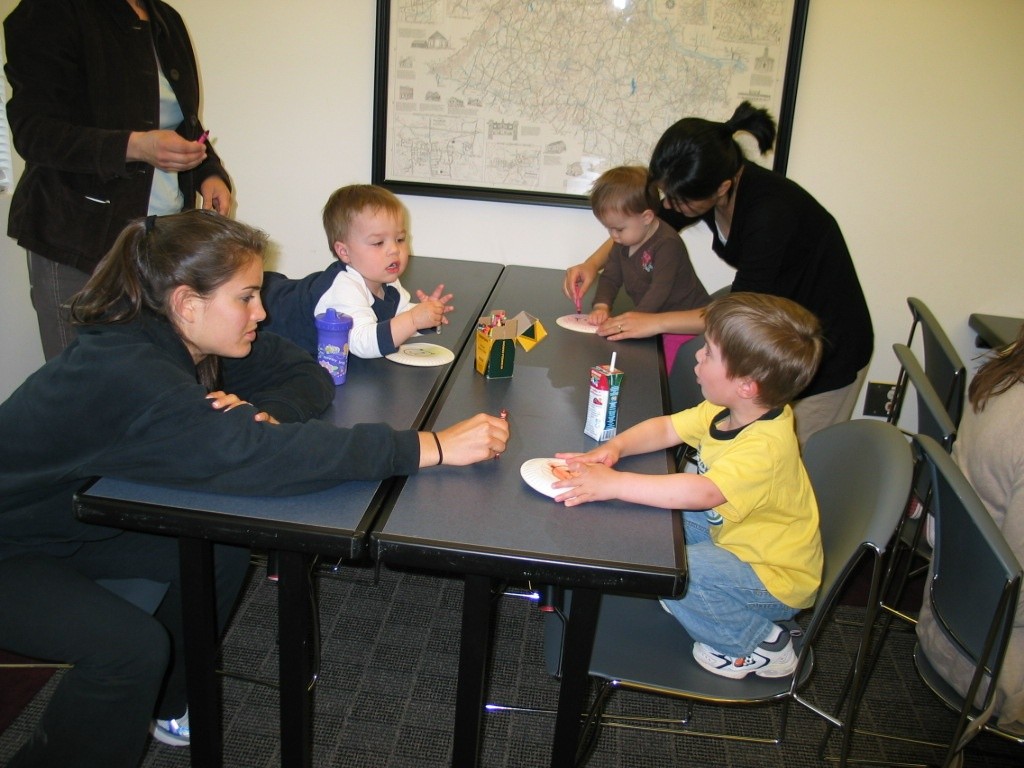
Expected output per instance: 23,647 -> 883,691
474,310 -> 549,379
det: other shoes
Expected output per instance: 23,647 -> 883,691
266,550 -> 281,586
150,704 -> 190,747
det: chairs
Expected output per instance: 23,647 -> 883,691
533,299 -> 1024,768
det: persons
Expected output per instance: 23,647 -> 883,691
0,209 -> 508,768
258,184 -> 454,365
917,324 -> 1024,736
0,0 -> 233,367
562,102 -> 875,457
550,289 -> 828,680
589,166 -> 712,381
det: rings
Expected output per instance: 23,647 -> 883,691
618,324 -> 622,332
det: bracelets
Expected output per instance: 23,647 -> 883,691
432,430 -> 443,466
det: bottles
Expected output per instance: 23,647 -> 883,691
315,307 -> 354,385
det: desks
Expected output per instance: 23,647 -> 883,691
372,265 -> 689,768
73,251 -> 504,768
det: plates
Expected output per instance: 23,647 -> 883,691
556,313 -> 601,333
384,343 -> 455,366
520,458 -> 577,500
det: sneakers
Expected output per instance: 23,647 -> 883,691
692,622 -> 803,680
